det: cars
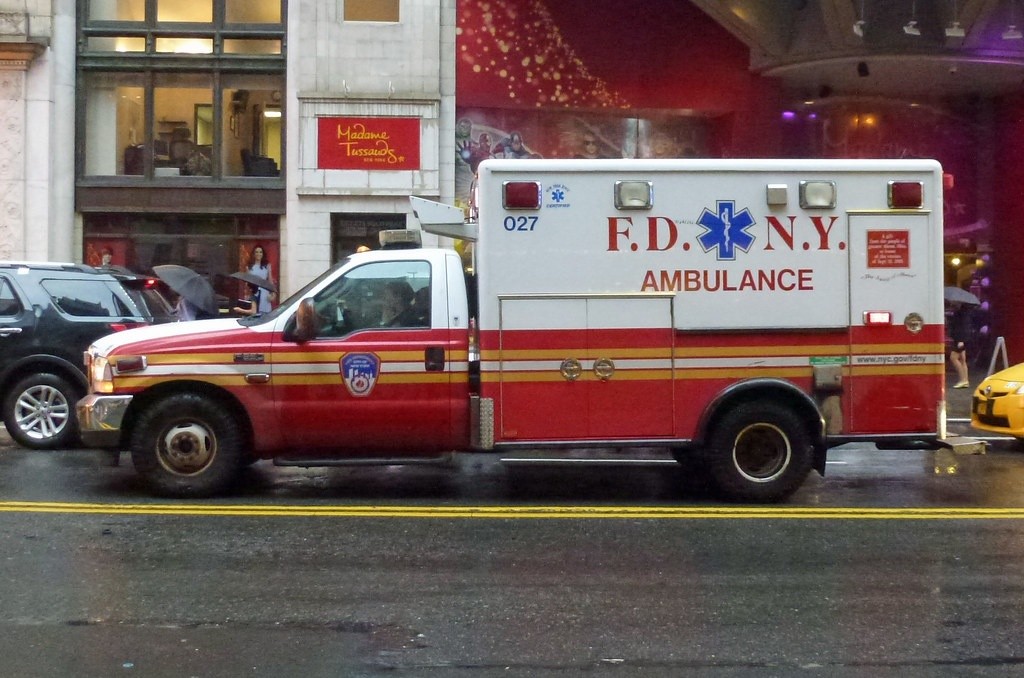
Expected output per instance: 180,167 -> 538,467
971,364 -> 1024,443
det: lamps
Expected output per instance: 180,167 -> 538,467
903,21 -> 921,35
1001,25 -> 1023,40
852,20 -> 866,37
945,21 -> 965,37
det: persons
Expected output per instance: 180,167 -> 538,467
573,131 -> 609,158
234,281 -> 261,317
245,245 -> 276,314
383,282 -> 419,327
950,302 -> 977,388
174,295 -> 201,321
214,272 -> 229,294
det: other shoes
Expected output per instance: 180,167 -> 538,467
953,381 -> 970,388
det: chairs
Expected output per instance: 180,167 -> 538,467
414,285 -> 430,326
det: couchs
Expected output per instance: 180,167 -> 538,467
239,149 -> 278,177
169,139 -> 212,175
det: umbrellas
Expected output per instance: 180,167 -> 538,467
231,273 -> 277,292
944,287 -> 980,304
153,265 -> 219,315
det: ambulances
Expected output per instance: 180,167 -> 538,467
72,157 -> 987,504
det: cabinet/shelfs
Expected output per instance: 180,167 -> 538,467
158,120 -> 188,135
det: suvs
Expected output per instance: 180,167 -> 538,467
0,261 -> 184,451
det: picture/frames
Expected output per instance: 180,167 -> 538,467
230,116 -> 235,131
234,113 -> 240,139
